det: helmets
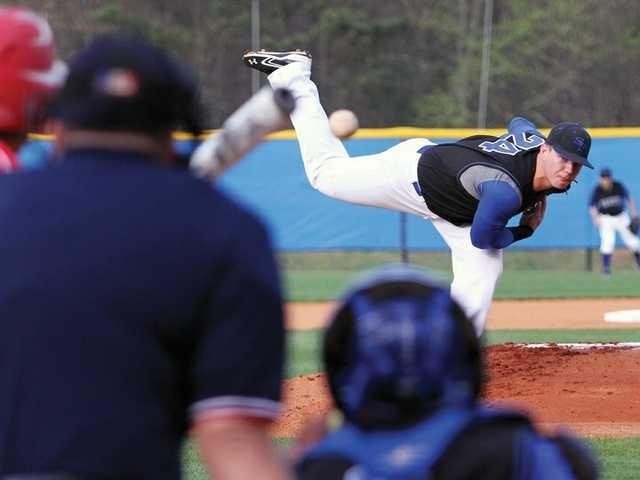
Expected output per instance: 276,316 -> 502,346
0,5 -> 70,135
324,281 -> 482,433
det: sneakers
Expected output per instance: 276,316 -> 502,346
239,47 -> 312,74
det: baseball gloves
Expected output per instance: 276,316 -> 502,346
628,214 -> 640,235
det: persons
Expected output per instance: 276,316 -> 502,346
241,45 -> 596,344
0,8 -> 68,174
278,261 -> 602,480
586,165 -> 640,278
0,25 -> 303,480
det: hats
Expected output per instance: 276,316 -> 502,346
546,122 -> 594,170
600,168 -> 609,176
62,31 -> 198,131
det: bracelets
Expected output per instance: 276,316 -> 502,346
505,224 -> 535,244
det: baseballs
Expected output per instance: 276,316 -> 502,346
328,109 -> 358,140
